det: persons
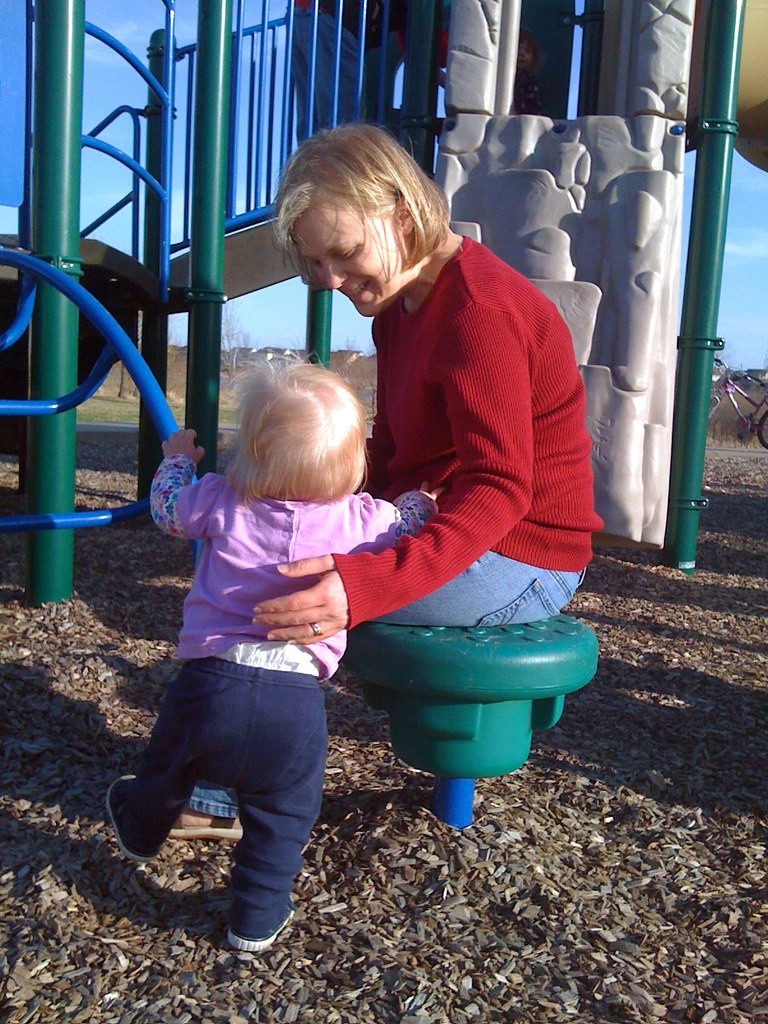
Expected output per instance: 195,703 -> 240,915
286,0 -> 541,150
168,126 -> 604,839
106,361 -> 446,950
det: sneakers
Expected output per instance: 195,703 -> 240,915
105,774 -> 160,862
228,895 -> 296,952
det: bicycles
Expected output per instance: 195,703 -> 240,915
708,357 -> 768,449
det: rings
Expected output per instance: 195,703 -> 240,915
310,621 -> 324,637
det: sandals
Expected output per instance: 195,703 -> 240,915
167,808 -> 244,842
